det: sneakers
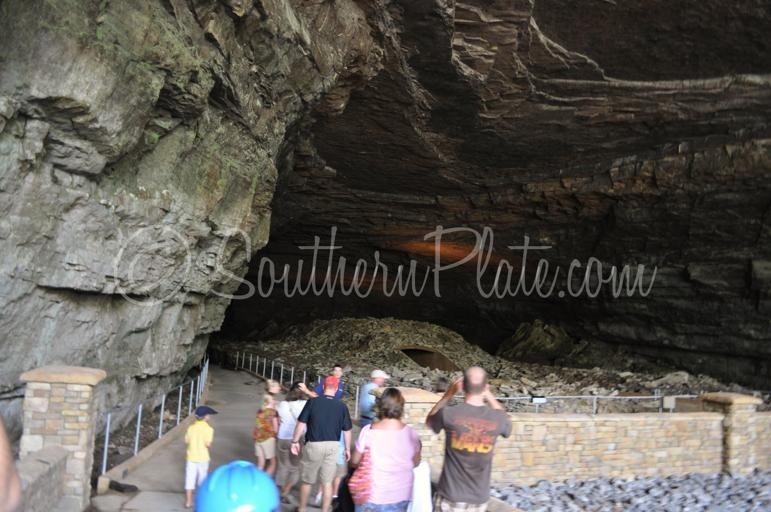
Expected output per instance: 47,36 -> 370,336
281,496 -> 292,503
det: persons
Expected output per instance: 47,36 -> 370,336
0,415 -> 23,511
180,404 -> 218,508
252,359 -> 422,511
425,365 -> 514,512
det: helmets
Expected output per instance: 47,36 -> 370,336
196,459 -> 280,512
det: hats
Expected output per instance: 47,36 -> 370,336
195,406 -> 218,418
371,370 -> 391,378
324,376 -> 338,387
